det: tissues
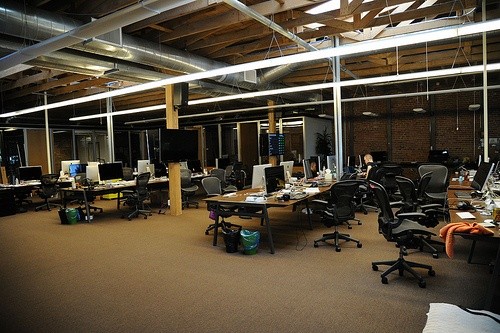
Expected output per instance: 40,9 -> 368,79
324,168 -> 332,180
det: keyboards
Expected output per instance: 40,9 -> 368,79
455,191 -> 472,199
290,193 -> 309,200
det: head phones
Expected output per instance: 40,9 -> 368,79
277,194 -> 290,201
456,200 -> 475,211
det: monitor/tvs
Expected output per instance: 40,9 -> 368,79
252,161 -> 294,195
19,165 -> 42,182
138,160 -> 151,176
370,150 -> 389,162
429,149 -> 449,163
60,159 -> 124,184
190,160 -> 203,173
470,161 -> 495,192
158,128 -> 199,163
303,155 -> 337,179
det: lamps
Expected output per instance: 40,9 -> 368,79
318,89 -> 333,119
468,75 -> 480,111
363,87 -> 378,117
413,82 -> 427,113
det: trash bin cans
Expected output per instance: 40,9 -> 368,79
66,208 -> 77,224
240,230 -> 259,254
223,229 -> 239,253
58,208 -> 68,224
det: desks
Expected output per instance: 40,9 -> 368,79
202,174 -> 361,254
60,174 -> 206,223
447,175 -> 500,263
0,180 -> 72,213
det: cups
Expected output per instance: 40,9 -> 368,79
493,208 -> 500,221
72,183 -> 76,188
455,172 -> 459,177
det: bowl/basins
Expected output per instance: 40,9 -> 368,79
467,170 -> 477,176
460,171 -> 468,175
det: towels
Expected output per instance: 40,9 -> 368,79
438,221 -> 494,260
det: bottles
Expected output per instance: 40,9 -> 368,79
460,176 -> 462,180
333,166 -> 336,179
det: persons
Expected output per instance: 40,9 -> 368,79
359,153 -> 378,198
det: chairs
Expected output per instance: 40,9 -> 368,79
121,166 -> 252,235
11,173 -> 102,216
311,164 -> 448,288
